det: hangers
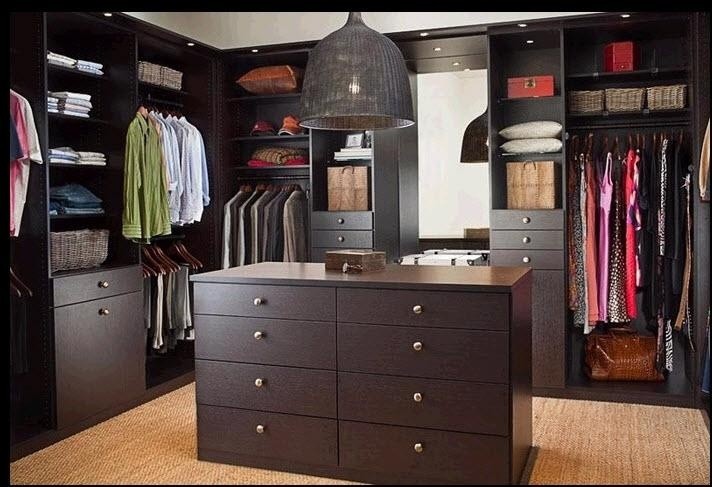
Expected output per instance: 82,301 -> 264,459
141,243 -> 204,278
240,178 -> 303,192
571,130 -> 690,155
136,98 -> 186,118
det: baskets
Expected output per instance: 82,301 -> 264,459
605,88 -> 646,111
50,230 -> 109,274
647,85 -> 687,110
138,62 -> 183,89
569,90 -> 604,112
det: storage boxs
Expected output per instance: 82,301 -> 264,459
507,76 -> 555,98
605,41 -> 640,72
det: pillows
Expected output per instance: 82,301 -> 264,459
499,139 -> 563,153
237,66 -> 305,95
498,119 -> 563,138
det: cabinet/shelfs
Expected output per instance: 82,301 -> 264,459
189,261 -> 532,486
12,12 -> 216,426
222,30 -> 400,263
490,12 -> 712,398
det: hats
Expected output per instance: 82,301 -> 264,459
250,120 -> 275,137
278,115 -> 302,136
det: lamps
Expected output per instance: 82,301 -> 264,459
296,12 -> 416,130
460,106 -> 489,163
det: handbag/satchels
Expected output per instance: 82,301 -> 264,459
506,161 -> 561,209
584,326 -> 666,381
326,166 -> 370,212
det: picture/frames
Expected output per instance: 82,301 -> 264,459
345,133 -> 364,148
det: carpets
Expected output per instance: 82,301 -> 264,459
10,380 -> 710,486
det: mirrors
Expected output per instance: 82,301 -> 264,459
417,68 -> 489,240
